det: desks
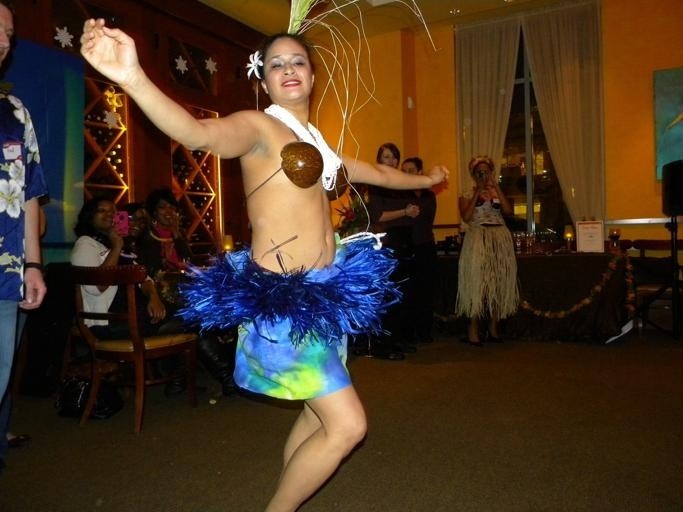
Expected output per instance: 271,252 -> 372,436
434,249 -> 628,343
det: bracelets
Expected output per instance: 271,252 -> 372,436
22,258 -> 42,271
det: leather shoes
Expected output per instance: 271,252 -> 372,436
217,357 -> 261,396
164,369 -> 187,397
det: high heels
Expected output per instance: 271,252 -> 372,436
467,330 -> 504,346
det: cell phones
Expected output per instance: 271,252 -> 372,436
113,211 -> 129,237
478,170 -> 488,183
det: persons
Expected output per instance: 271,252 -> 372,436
1,0 -> 60,469
458,153 -> 514,348
71,188 -> 219,418
79,17 -> 450,512
363,144 -> 422,361
401,156 -> 437,344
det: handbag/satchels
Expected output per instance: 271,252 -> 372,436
55,378 -> 124,420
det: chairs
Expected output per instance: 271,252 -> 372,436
604,239 -> 682,342
63,261 -> 202,435
